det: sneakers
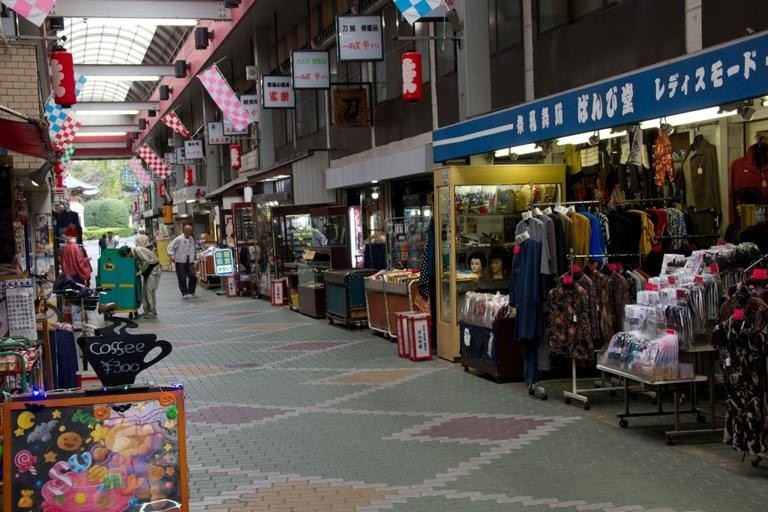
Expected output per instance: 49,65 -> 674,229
136,311 -> 157,318
183,293 -> 196,299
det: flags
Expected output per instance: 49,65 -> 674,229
160,109 -> 189,138
133,144 -> 172,182
195,64 -> 249,130
42,65 -> 85,170
127,155 -> 154,190
392,0 -> 450,25
3,1 -> 56,26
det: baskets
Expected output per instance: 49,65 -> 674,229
62,292 -> 100,314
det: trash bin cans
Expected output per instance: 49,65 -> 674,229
271,280 -> 285,307
395,309 -> 433,362
225,273 -> 238,298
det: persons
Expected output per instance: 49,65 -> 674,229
98,231 -> 120,255
467,250 -> 487,275
488,245 -> 506,281
165,224 -> 197,298
134,224 -> 157,253
120,245 -> 162,319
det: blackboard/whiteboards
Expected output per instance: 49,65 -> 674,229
0,389 -> 191,512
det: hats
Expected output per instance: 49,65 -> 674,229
119,246 -> 128,256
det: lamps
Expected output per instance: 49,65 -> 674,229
126,27 -> 215,148
508,116 -> 674,162
716,98 -> 756,122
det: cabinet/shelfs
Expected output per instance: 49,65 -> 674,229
432,163 -> 571,364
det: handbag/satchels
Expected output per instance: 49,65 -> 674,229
142,264 -> 153,278
52,272 -> 97,310
